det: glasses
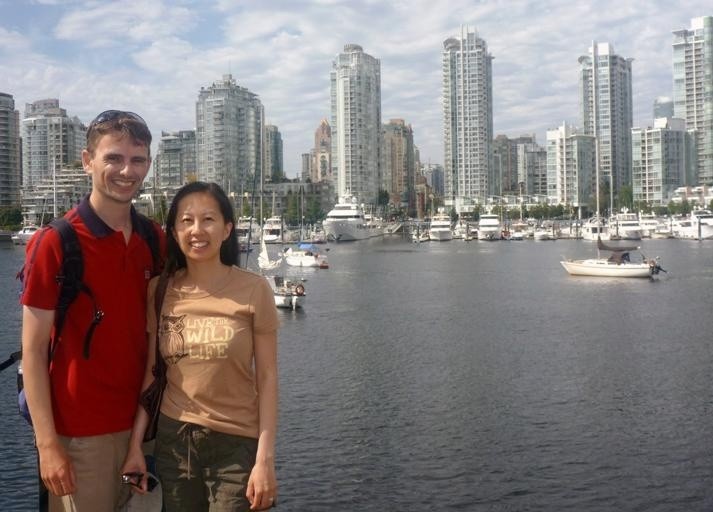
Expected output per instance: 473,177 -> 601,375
83,109 -> 149,140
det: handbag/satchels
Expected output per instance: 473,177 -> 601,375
135,359 -> 169,443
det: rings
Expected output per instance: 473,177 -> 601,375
270,497 -> 274,501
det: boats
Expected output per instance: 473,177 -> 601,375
10,224 -> 41,246
322,187 -> 400,241
235,110 -> 329,315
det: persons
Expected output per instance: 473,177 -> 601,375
123,181 -> 278,512
19,109 -> 176,512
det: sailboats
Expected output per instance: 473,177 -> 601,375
557,139 -> 668,279
